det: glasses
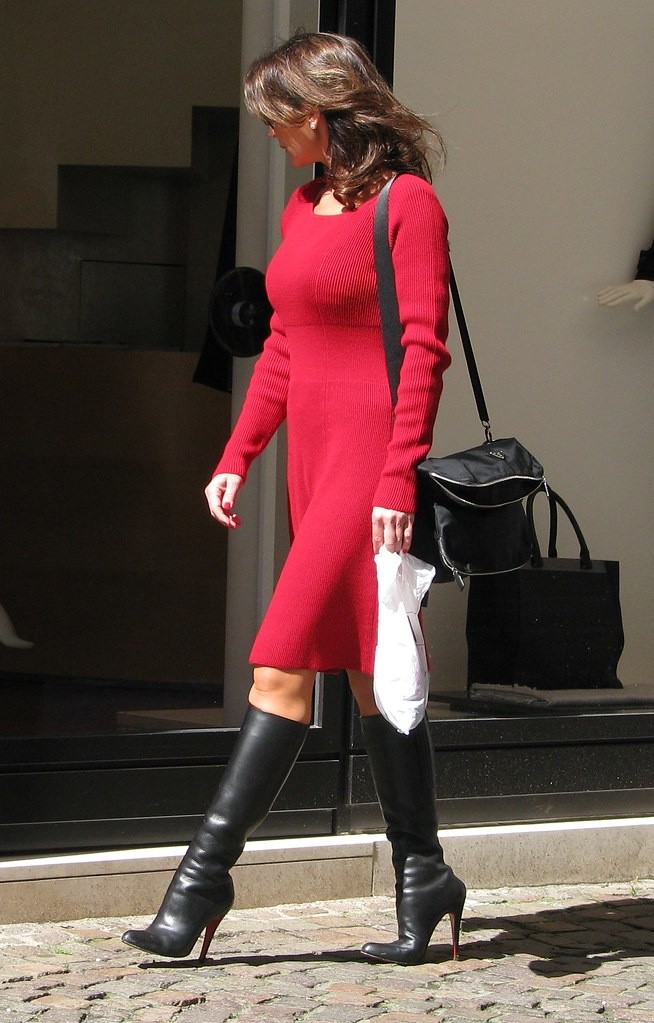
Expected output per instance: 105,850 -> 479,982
261,115 -> 276,129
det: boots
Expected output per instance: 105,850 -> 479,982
360,710 -> 467,965
121,702 -> 310,963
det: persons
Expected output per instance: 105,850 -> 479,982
597,237 -> 654,312
120,30 -> 468,966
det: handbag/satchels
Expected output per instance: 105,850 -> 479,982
465,482 -> 624,689
373,543 -> 437,735
408,436 -> 544,592
